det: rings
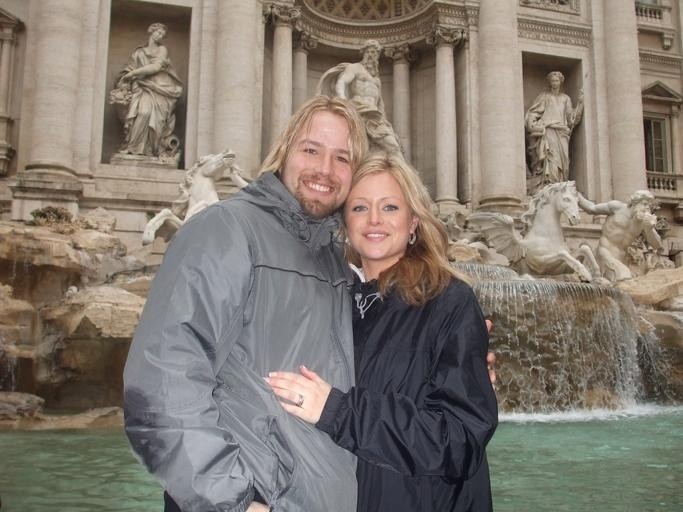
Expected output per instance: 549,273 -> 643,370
294,395 -> 303,409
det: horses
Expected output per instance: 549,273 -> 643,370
522,180 -> 602,283
141,147 -> 237,246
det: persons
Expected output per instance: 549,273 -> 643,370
523,69 -> 582,185
316,38 -> 403,160
121,92 -> 499,511
576,187 -> 663,286
106,21 -> 185,154
257,152 -> 499,511
